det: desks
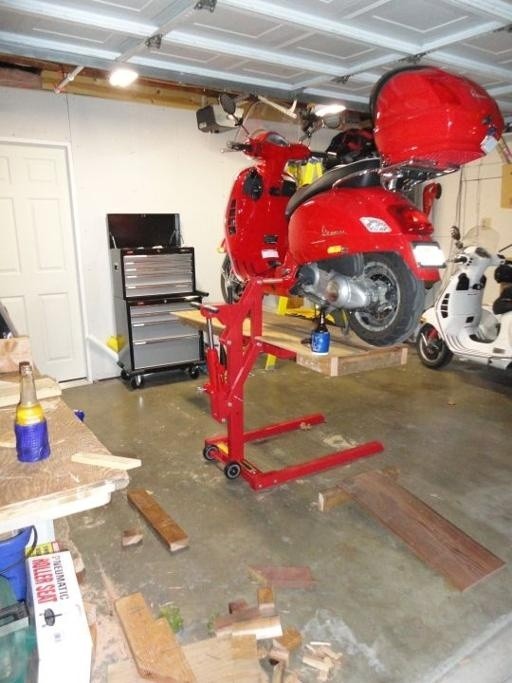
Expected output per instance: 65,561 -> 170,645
0,356 -> 130,578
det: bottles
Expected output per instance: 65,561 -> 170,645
15,364 -> 51,462
311,306 -> 329,352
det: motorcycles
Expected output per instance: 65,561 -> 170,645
217,63 -> 505,352
414,224 -> 512,372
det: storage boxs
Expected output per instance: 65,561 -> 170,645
24,543 -> 93,683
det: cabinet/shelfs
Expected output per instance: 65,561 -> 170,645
109,248 -> 210,390
